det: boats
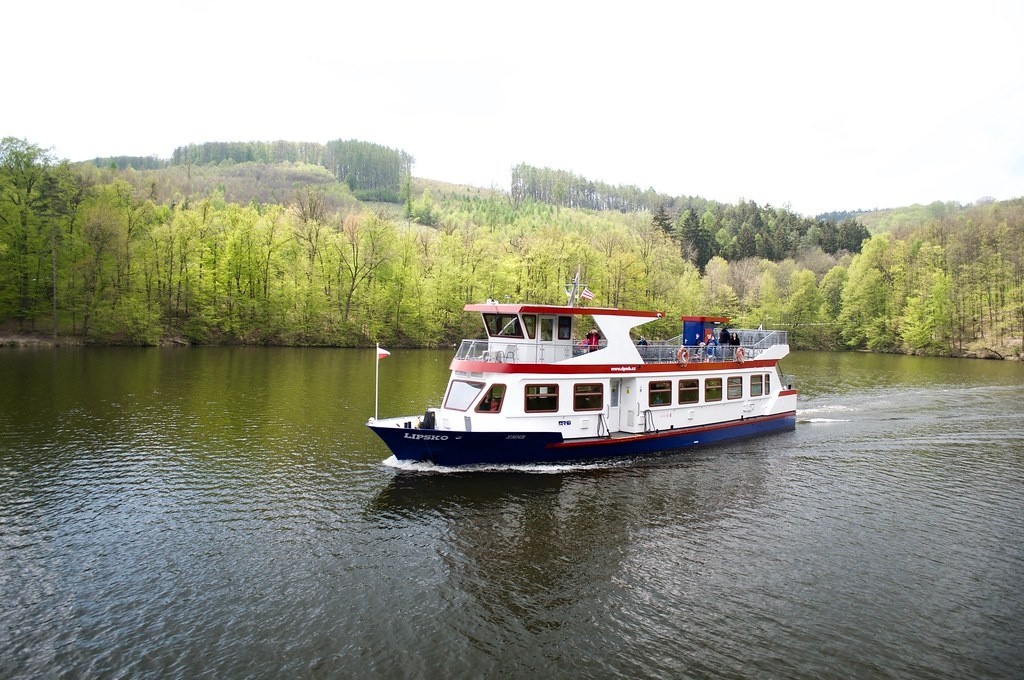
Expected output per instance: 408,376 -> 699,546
364,263 -> 798,467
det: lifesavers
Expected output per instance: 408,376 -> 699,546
677,348 -> 689,363
736,347 -> 745,359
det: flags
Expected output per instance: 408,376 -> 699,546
580,288 -> 595,300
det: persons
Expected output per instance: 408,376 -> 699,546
480,391 -> 499,412
718,326 -> 731,356
693,332 -> 719,363
584,326 -> 601,353
636,335 -> 648,363
729,333 -> 740,357
